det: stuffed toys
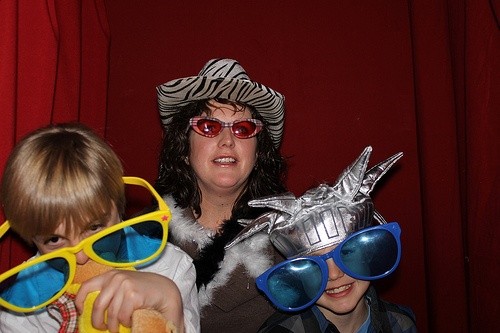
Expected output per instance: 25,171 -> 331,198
46,258 -> 176,333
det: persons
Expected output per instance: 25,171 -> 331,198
0,122 -> 202,333
225,144 -> 418,333
134,58 -> 290,333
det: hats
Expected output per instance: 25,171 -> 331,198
224,145 -> 404,260
156,58 -> 285,150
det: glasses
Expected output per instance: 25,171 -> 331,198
184,116 -> 264,139
0,176 -> 172,313
255,222 -> 401,311
